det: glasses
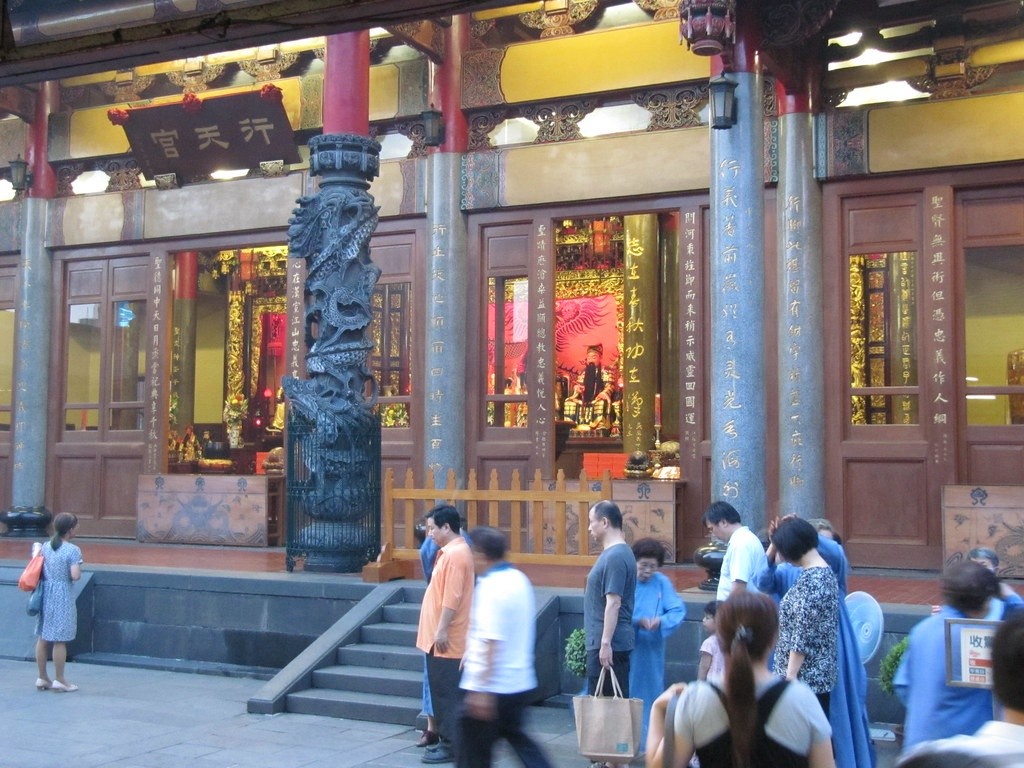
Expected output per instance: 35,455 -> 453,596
637,562 -> 658,569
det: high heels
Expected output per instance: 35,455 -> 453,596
52,680 -> 79,691
36,678 -> 52,691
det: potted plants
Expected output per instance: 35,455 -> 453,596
878,636 -> 907,747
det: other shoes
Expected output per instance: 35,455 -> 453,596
585,760 -> 607,768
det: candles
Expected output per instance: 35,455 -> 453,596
655,393 -> 661,426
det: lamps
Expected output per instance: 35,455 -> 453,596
708,69 -> 737,130
9,153 -> 32,195
421,103 -> 445,147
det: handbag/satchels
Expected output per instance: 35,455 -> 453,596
18,544 -> 43,592
26,581 -> 42,617
573,666 -> 643,757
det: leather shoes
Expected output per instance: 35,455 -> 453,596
416,732 -> 439,745
422,745 -> 455,762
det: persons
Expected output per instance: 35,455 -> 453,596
757,513 -> 878,768
702,501 -> 772,602
563,344 -> 616,432
453,524 -> 553,768
627,538 -> 687,761
35,512 -> 84,692
416,502 -> 475,764
690,601 -> 728,768
169,422 -> 214,464
894,609 -> 1024,767
645,588 -> 836,768
893,560 -> 1024,753
930,549 -> 1001,617
583,499 -> 637,768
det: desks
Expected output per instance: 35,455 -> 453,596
136,474 -> 284,547
940,483 -> 1024,579
527,479 -> 686,563
